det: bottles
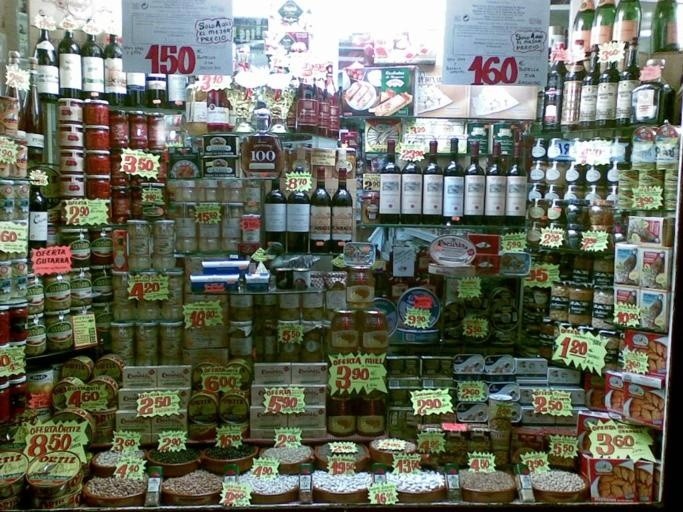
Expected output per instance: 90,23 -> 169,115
0,0 -> 683,257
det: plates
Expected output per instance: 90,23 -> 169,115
586,381 -> 664,501
622,335 -> 668,371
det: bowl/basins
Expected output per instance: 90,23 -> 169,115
83,440 -> 591,505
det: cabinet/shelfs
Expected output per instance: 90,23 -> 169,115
0,1 -> 683,510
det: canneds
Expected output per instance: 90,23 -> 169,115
361,192 -> 377,223
525,136 -> 677,369
313,149 -> 354,179
1,354 -> 125,505
0,97 -> 28,310
128,72 -> 187,109
113,221 -> 174,273
60,97 -> 166,220
114,275 -> 182,365
29,229 -> 112,351
188,357 -> 253,440
330,395 -> 384,435
169,180 -> 260,253
